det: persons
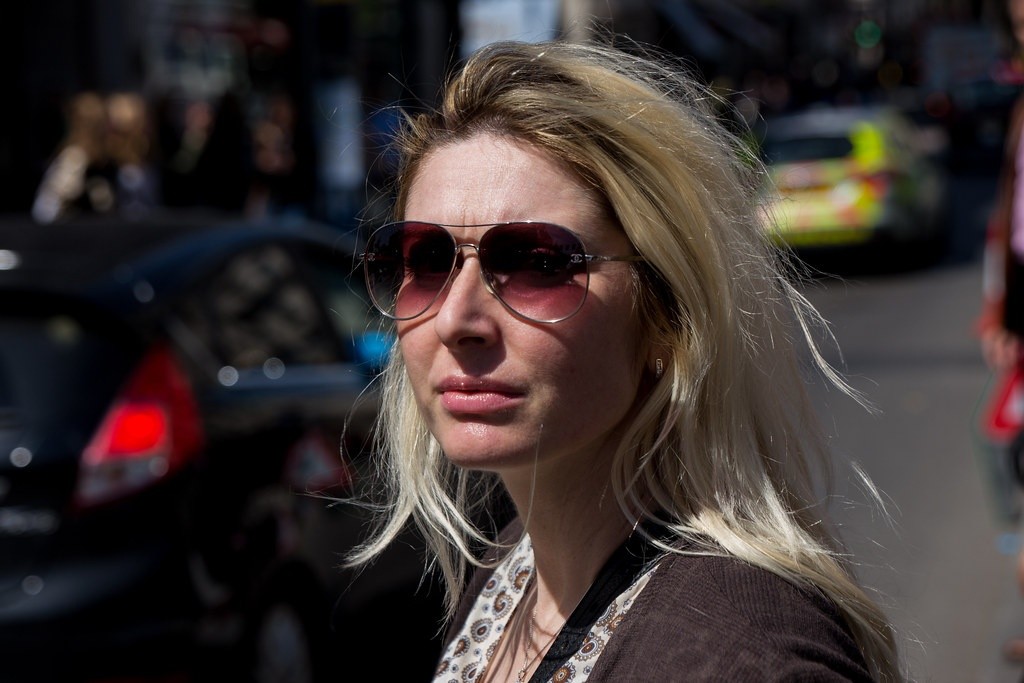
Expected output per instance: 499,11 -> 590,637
972,95 -> 1024,662
34,90 -> 308,225
398,39 -> 899,682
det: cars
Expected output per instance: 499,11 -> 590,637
752,110 -> 953,277
0,221 -> 523,683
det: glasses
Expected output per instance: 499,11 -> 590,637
359,221 -> 645,325
